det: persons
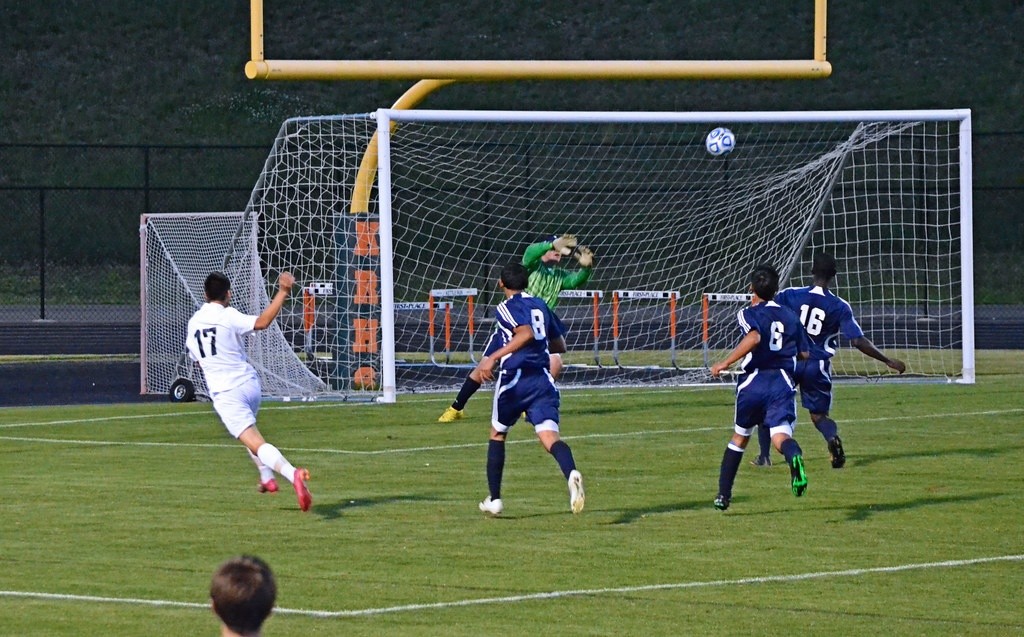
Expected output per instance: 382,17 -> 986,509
711,252 -> 906,511
185,272 -> 313,512
438,233 -> 593,422
478,264 -> 586,514
211,555 -> 276,637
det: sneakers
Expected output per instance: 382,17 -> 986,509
787,452 -> 808,498
478,495 -> 504,515
291,466 -> 312,512
827,435 -> 846,469
255,478 -> 279,492
567,470 -> 585,513
749,454 -> 773,466
437,406 -> 465,423
714,494 -> 731,510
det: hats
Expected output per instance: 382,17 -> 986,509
812,251 -> 839,276
539,235 -> 557,243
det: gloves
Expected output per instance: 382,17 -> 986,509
552,233 -> 577,255
574,244 -> 595,266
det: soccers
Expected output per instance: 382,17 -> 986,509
707,127 -> 736,156
